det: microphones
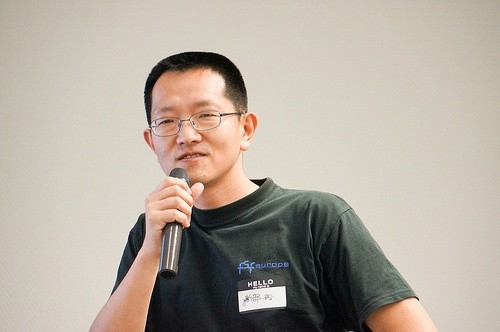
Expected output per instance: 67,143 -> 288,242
159,168 -> 190,278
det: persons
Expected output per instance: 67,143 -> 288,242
86,51 -> 437,332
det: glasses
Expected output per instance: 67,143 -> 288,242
147,110 -> 239,137
